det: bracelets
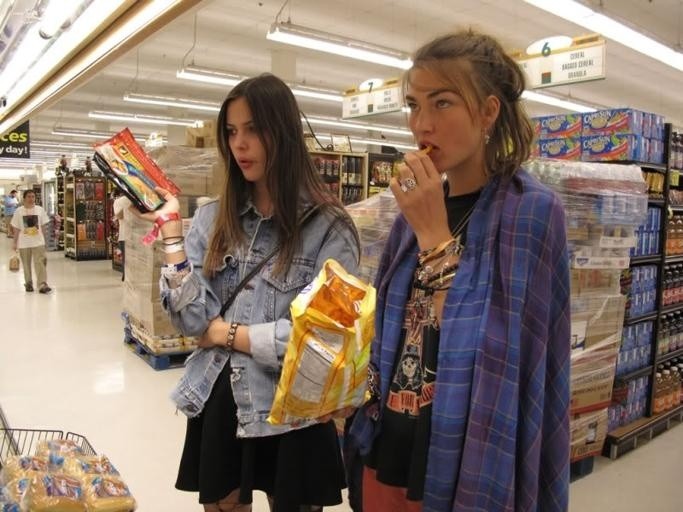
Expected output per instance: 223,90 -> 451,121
225,322 -> 239,352
414,239 -> 463,296
142,213 -> 190,280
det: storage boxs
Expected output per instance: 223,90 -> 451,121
118,120 -> 237,357
333,162 -> 650,464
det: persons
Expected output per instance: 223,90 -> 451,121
128,72 -> 362,512
112,188 -> 133,281
9,190 -> 52,293
4,190 -> 19,237
343,31 -> 568,512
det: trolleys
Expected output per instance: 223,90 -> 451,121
0,424 -> 132,512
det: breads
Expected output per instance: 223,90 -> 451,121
0,438 -> 136,512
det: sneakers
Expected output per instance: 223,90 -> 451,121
24,283 -> 52,294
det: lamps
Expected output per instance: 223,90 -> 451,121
2,140 -> 95,161
284,82 -> 345,102
314,133 -> 419,151
266,22 -> 414,71
123,93 -> 222,113
88,110 -> 204,129
300,115 -> 414,136
51,127 -> 167,143
176,66 -> 251,87
358,78 -> 386,94
525,1 -> 682,71
519,88 -> 614,114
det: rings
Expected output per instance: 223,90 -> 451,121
401,177 -> 418,193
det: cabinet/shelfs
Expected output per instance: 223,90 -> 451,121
295,151 -> 404,205
608,115 -> 682,464
35,173 -> 124,273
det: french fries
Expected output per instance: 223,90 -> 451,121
417,146 -> 432,156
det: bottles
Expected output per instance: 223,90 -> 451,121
651,131 -> 683,415
58,180 -> 64,248
337,155 -> 364,207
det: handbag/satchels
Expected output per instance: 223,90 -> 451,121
9,252 -> 20,271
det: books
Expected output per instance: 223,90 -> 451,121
93,128 -> 181,214
